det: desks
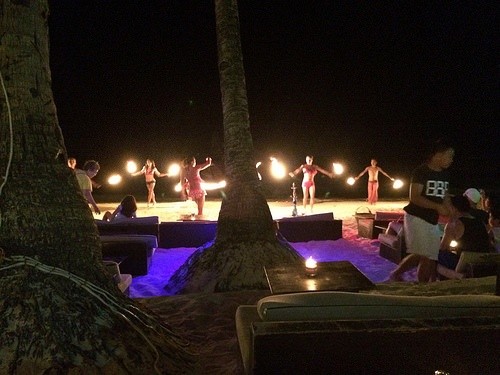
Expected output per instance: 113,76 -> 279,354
262,261 -> 377,296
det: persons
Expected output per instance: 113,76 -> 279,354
384,216 -> 407,238
355,158 -> 395,205
390,137 -> 455,285
289,154 -> 333,213
74,160 -> 101,215
68,156 -> 76,169
439,188 -> 496,271
180,154 -> 212,215
102,195 -> 137,222
131,158 -> 168,207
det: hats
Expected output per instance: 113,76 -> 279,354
465,188 -> 480,203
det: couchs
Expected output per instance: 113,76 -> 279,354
357,211 -> 500,281
235,291 -> 500,375
94,216 -> 218,292
277,211 -> 343,244
